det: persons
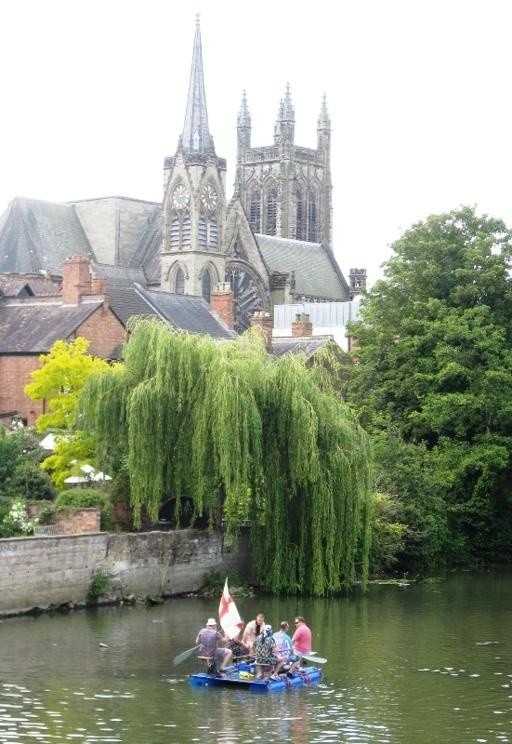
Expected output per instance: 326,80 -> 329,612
196,617 -> 231,671
239,612 -> 268,654
250,626 -> 285,681
273,621 -> 302,677
292,616 -> 312,658
222,631 -> 252,666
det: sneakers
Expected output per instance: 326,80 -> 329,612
270,674 -> 282,681
287,671 -> 295,679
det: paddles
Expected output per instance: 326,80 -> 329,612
293,652 -> 327,664
173,629 -> 224,665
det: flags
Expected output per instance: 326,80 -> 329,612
218,579 -> 243,640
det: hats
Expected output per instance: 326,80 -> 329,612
206,618 -> 217,626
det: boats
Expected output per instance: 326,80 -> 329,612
187,658 -> 322,693
